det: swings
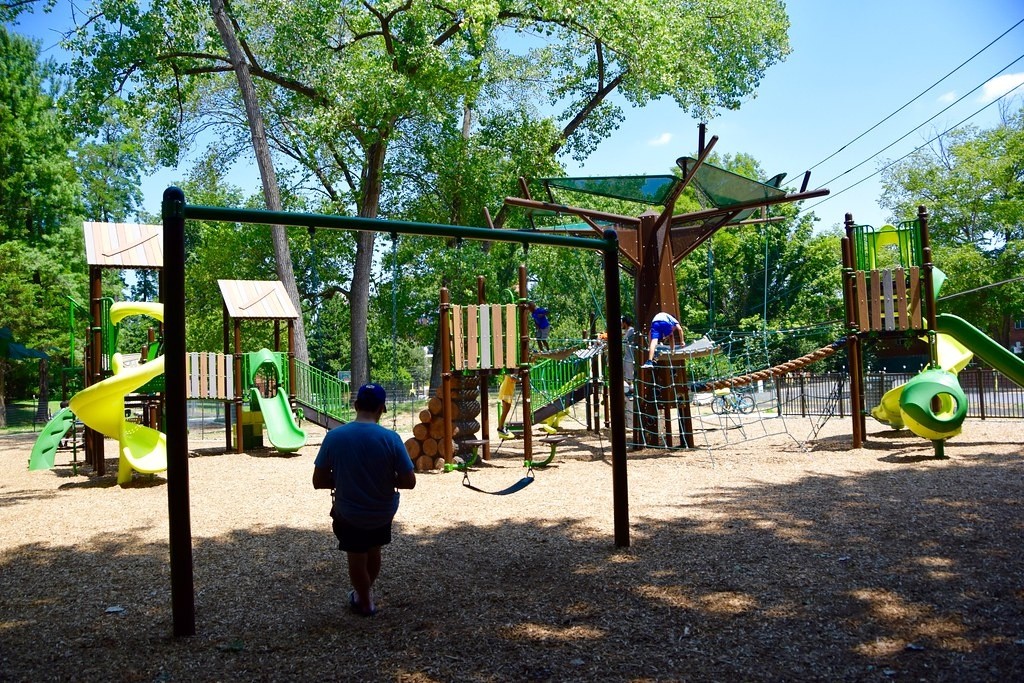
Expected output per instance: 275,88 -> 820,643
458,239 -> 533,497
306,227 -> 401,550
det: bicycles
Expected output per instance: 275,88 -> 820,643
710,382 -> 760,415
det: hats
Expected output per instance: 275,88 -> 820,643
357,383 -> 387,413
621,315 -> 633,325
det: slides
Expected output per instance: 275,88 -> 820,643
242,387 -> 310,456
872,317 -> 974,432
65,351 -> 166,483
936,313 -> 1024,389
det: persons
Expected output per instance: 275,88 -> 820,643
311,383 -> 416,616
497,374 -> 518,436
620,315 -> 636,395
526,300 -> 550,352
641,311 -> 685,368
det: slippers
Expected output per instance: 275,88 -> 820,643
348,590 -> 376,615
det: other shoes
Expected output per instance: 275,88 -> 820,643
641,360 -> 654,369
497,427 -> 508,436
